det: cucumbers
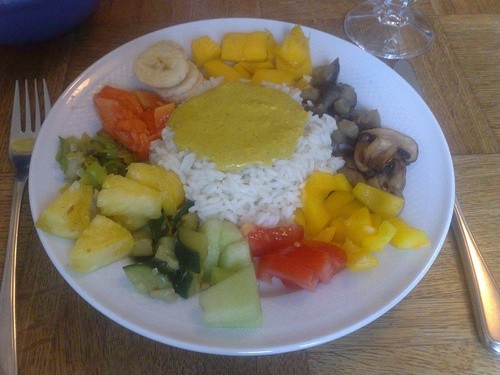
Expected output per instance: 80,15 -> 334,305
123,200 -> 263,328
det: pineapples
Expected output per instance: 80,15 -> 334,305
34,163 -> 186,273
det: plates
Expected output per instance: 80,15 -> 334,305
29,17 -> 456,357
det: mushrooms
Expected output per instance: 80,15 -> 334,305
302,59 -> 420,198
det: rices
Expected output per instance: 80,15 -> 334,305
147,75 -> 346,234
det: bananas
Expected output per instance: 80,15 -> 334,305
134,43 -> 208,103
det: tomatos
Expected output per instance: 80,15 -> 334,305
246,225 -> 348,290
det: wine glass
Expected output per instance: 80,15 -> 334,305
345,0 -> 437,59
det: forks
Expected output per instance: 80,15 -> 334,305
0,80 -> 53,375
